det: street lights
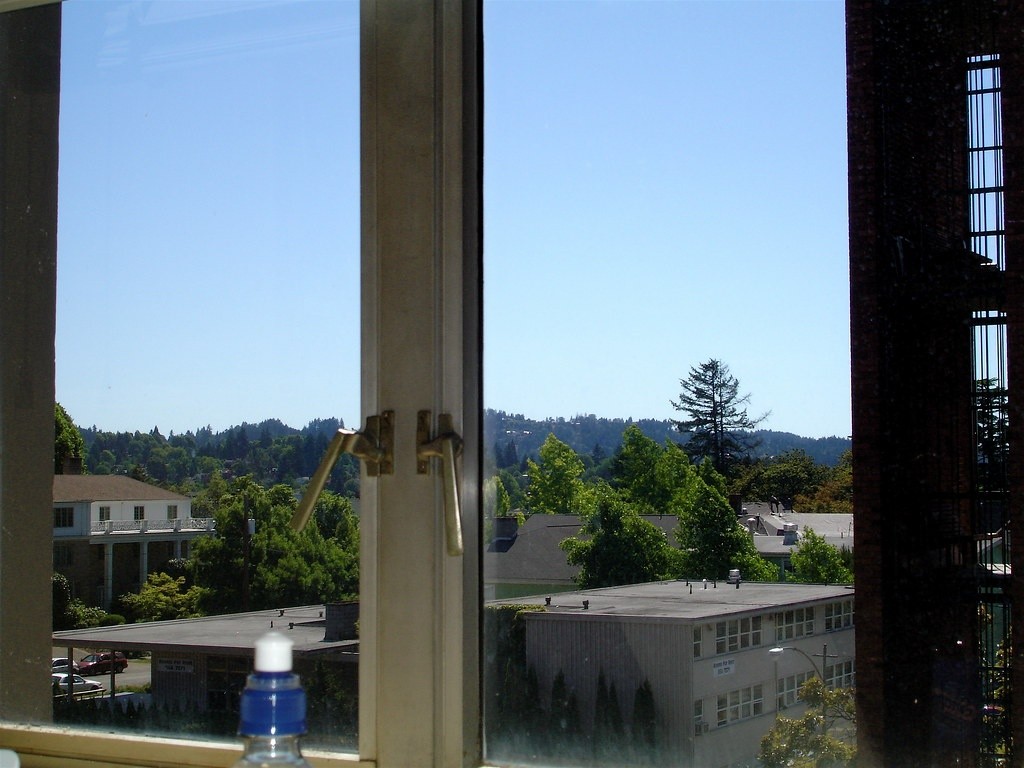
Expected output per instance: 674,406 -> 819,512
768,642 -> 839,737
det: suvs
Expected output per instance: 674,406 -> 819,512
52,657 -> 80,673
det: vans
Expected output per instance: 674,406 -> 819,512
77,651 -> 128,674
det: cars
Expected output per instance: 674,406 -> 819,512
52,673 -> 102,694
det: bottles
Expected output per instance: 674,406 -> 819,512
233,633 -> 310,768
